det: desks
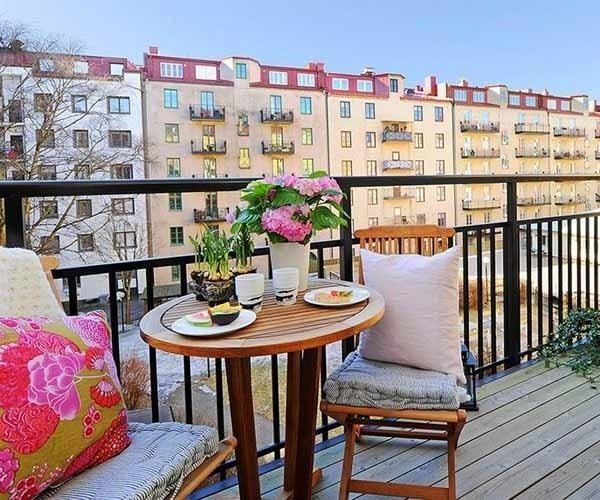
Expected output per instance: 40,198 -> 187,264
138,274 -> 387,500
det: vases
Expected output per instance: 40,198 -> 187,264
270,241 -> 311,292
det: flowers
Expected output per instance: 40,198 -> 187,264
229,169 -> 352,245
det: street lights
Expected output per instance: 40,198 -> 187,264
483,256 -> 490,306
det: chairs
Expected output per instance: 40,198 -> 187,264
319,224 -> 467,500
1,243 -> 238,500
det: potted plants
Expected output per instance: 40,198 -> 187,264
184,221 -> 257,306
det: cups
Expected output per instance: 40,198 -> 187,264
235,273 -> 264,313
273,268 -> 299,305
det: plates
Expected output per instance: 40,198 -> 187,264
303,286 -> 369,307
171,308 -> 257,337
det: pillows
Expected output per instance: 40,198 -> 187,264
0,309 -> 135,500
354,246 -> 470,386
